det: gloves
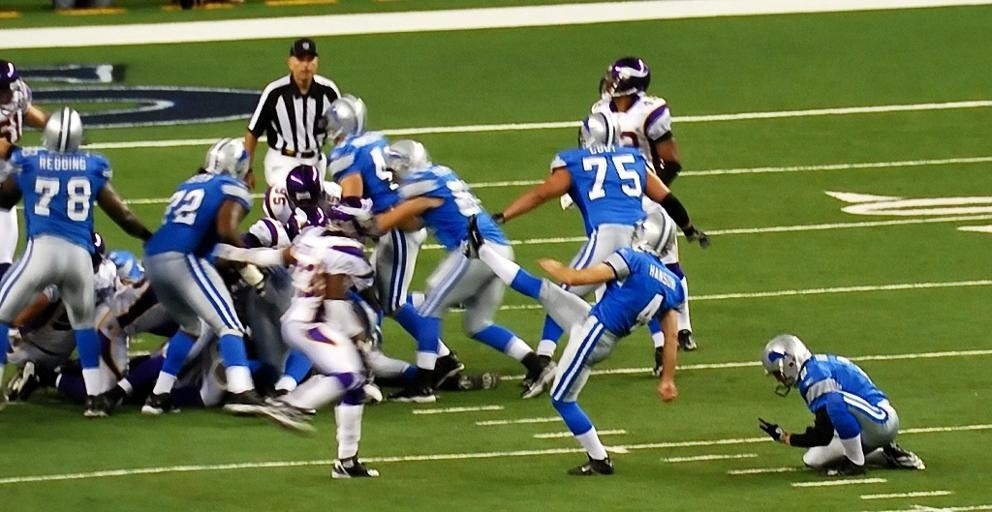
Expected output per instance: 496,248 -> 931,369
757,416 -> 787,443
684,226 -> 711,248
254,275 -> 274,300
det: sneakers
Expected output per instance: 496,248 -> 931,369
653,347 -> 667,376
385,354 -> 495,402
4,359 -> 38,403
882,438 -> 925,470
824,454 -> 866,476
223,386 -> 317,433
569,453 -> 612,475
519,360 -> 558,399
81,393 -> 115,418
331,455 -> 379,478
678,328 -> 697,352
141,390 -> 181,415
462,212 -> 485,260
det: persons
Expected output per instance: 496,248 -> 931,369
1,40 -> 708,479
758,333 -> 927,474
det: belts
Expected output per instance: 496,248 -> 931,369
282,149 -> 316,160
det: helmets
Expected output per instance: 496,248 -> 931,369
598,55 -> 651,102
633,210 -> 679,258
576,111 -> 618,149
287,206 -> 326,235
328,197 -> 372,243
92,231 -> 107,269
762,334 -> 811,399
382,139 -> 430,179
41,107 -> 83,153
324,93 -> 367,139
287,164 -> 321,207
206,136 -> 250,179
0,59 -> 26,114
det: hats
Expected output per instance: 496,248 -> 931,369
290,39 -> 319,60
